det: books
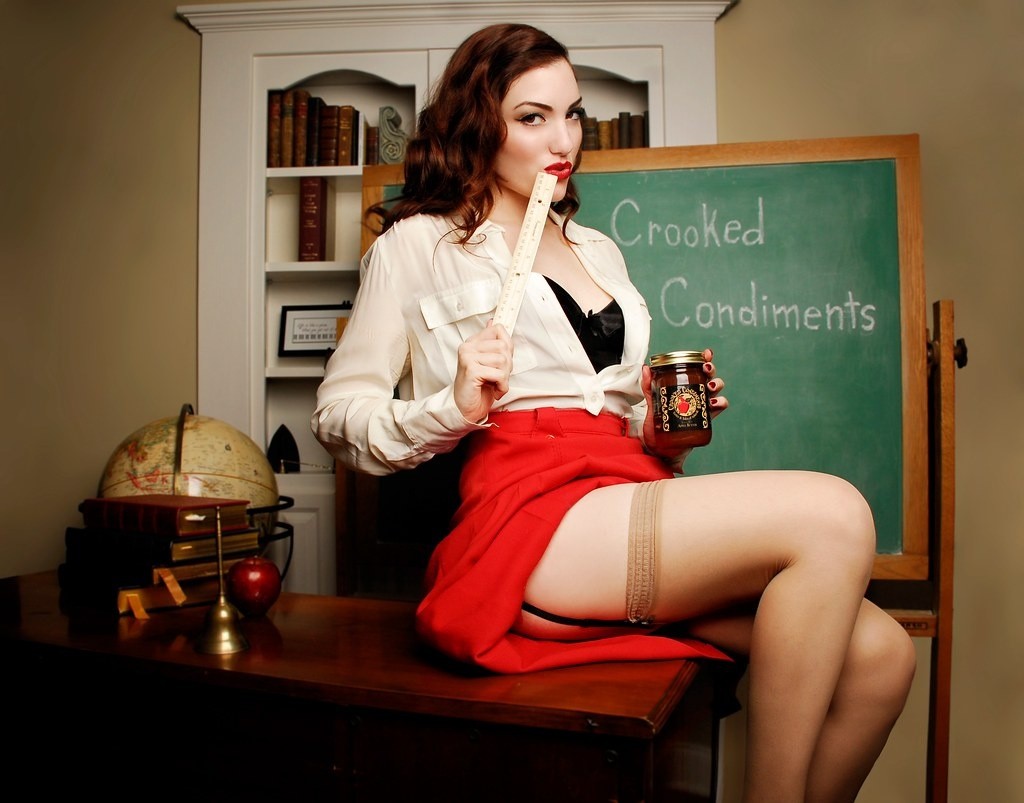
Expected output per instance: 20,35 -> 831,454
59,494 -> 258,615
300,177 -> 327,260
582,111 -> 649,151
267,90 -> 378,166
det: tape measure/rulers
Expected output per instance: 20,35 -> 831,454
490,169 -> 559,336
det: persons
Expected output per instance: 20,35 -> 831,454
309,23 -> 917,803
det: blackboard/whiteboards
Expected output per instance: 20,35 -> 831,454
348,136 -> 928,595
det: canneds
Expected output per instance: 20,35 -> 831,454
648,350 -> 712,448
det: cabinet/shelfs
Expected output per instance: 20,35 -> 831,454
176,0 -> 731,598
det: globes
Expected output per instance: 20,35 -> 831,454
92,399 -> 282,548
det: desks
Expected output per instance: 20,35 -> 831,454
0,570 -> 723,803
